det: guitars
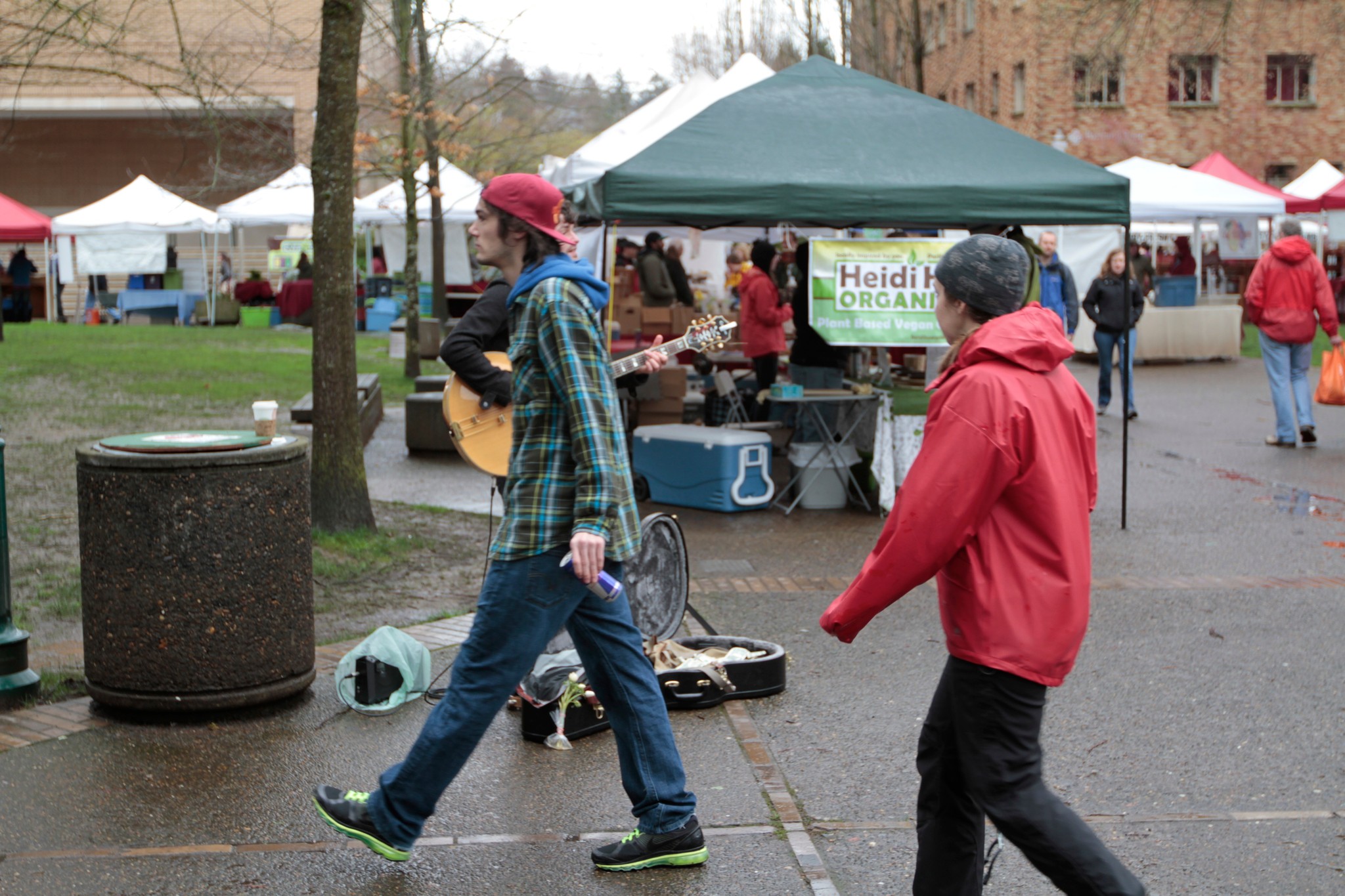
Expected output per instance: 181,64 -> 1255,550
442,314 -> 739,480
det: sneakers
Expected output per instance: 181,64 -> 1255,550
310,783 -> 411,862
592,814 -> 710,870
1265,435 -> 1296,447
1300,425 -> 1316,442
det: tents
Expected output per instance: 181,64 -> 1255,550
0,50 -> 1345,532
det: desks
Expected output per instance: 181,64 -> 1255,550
761,384 -> 883,519
116,291 -> 202,331
0,274 -> 46,318
97,293 -> 122,323
1074,295 -> 1245,363
856,374 -> 941,488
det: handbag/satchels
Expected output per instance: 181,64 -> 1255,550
1312,343 -> 1345,406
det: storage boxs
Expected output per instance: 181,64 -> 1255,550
600,260 -> 750,361
130,249 -> 183,290
1150,275 -> 1196,308
632,365 -> 688,399
631,422 -> 776,515
637,399 -> 683,424
196,271 -> 482,331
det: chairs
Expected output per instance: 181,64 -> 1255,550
712,368 -> 783,438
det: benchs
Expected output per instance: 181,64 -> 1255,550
292,371 -> 384,450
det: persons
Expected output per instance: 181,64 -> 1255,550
308,169 -> 711,873
819,228 -> 1157,896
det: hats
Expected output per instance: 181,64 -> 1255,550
935,232 -> 1027,318
752,237 -> 777,275
1174,236 -> 1188,254
480,172 -> 576,247
645,231 -> 668,243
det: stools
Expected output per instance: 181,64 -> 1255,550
386,313 -> 462,363
404,391 -> 460,460
414,373 -> 454,392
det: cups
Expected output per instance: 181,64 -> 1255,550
251,399 -> 278,438
